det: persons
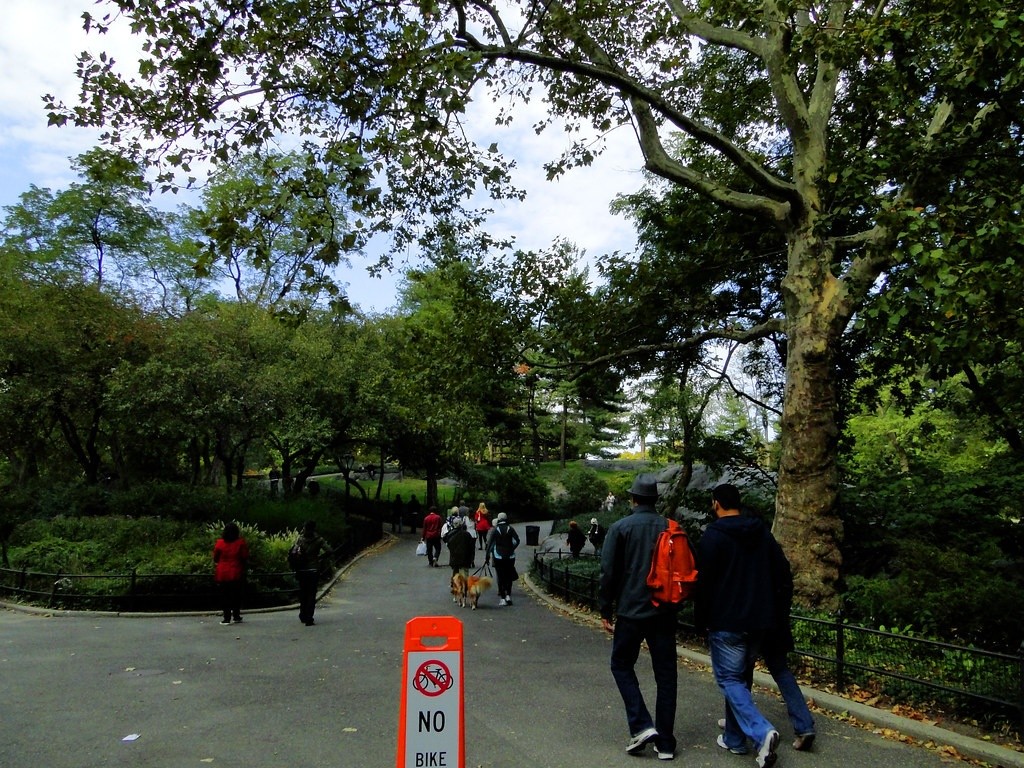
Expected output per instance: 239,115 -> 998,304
407,494 -> 421,535
695,484 -> 817,768
269,466 -> 280,493
368,461 -> 377,474
443,518 -> 475,588
459,500 -> 466,517
590,517 -> 607,554
441,506 -> 462,531
212,523 -> 250,625
598,474 -> 679,759
359,464 -> 362,469
566,521 -> 587,559
392,495 -> 405,533
487,512 -> 520,606
422,506 -> 443,567
601,491 -> 615,511
292,520 -> 327,629
463,508 -> 477,568
474,502 -> 492,550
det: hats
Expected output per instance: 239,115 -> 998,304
492,519 -> 498,526
588,518 -> 599,534
626,474 -> 659,497
570,521 -> 578,528
498,513 -> 507,520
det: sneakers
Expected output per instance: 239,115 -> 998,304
233,617 -> 243,623
756,729 -> 780,768
499,599 -> 507,606
505,595 -> 514,605
220,620 -> 230,625
625,728 -> 659,754
717,734 -> 746,753
653,745 -> 674,759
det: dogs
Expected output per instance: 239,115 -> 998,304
450,573 -> 493,611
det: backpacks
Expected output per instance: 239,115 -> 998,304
288,535 -> 320,571
639,518 -> 698,614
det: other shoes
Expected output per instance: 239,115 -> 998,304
433,557 -> 439,567
718,718 -> 726,727
793,734 -> 815,750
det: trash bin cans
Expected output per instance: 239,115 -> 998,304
526,525 -> 540,545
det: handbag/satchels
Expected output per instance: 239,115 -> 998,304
415,538 -> 427,555
512,567 -> 519,581
462,521 -> 467,531
440,522 -> 453,538
477,512 -> 481,521
